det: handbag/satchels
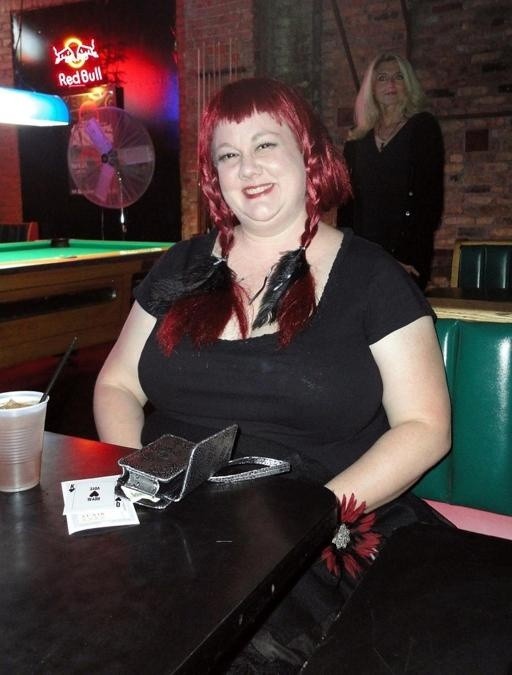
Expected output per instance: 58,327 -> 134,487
114,424 -> 238,510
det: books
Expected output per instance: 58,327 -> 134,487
58,474 -> 141,536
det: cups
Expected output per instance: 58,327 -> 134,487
0,389 -> 49,493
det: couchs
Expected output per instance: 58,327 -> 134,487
412,310 -> 511,542
450,241 -> 510,302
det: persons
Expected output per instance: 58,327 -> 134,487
335,52 -> 444,292
96,79 -> 452,647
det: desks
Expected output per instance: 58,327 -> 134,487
0,431 -> 342,674
0,239 -> 177,367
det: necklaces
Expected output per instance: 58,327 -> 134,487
378,119 -> 405,151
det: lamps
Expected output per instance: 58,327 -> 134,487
1,0 -> 70,126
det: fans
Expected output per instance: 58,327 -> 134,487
68,108 -> 154,214
